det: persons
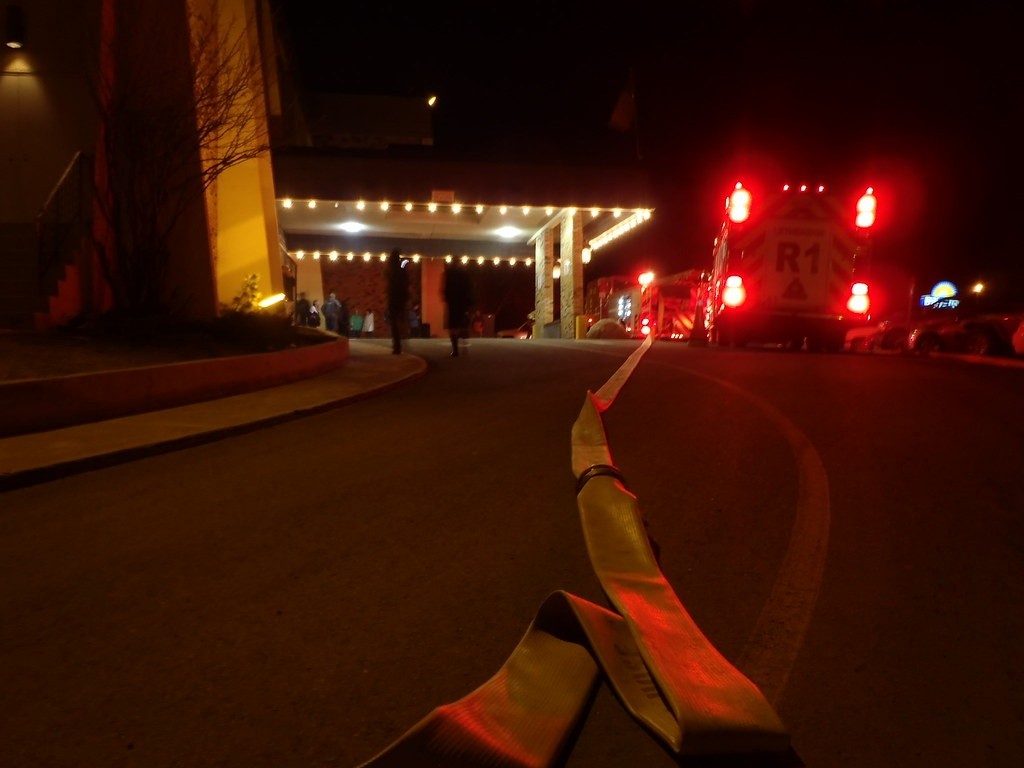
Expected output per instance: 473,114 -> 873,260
383,247 -> 408,356
439,255 -> 475,360
409,302 -> 424,339
472,308 -> 485,338
349,309 -> 363,339
362,308 -> 374,339
296,292 -> 349,336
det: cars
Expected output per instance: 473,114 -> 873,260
842,297 -> 1024,356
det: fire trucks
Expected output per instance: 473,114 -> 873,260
696,182 -> 866,355
636,278 -> 702,341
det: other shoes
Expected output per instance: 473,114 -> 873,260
450,352 -> 460,358
